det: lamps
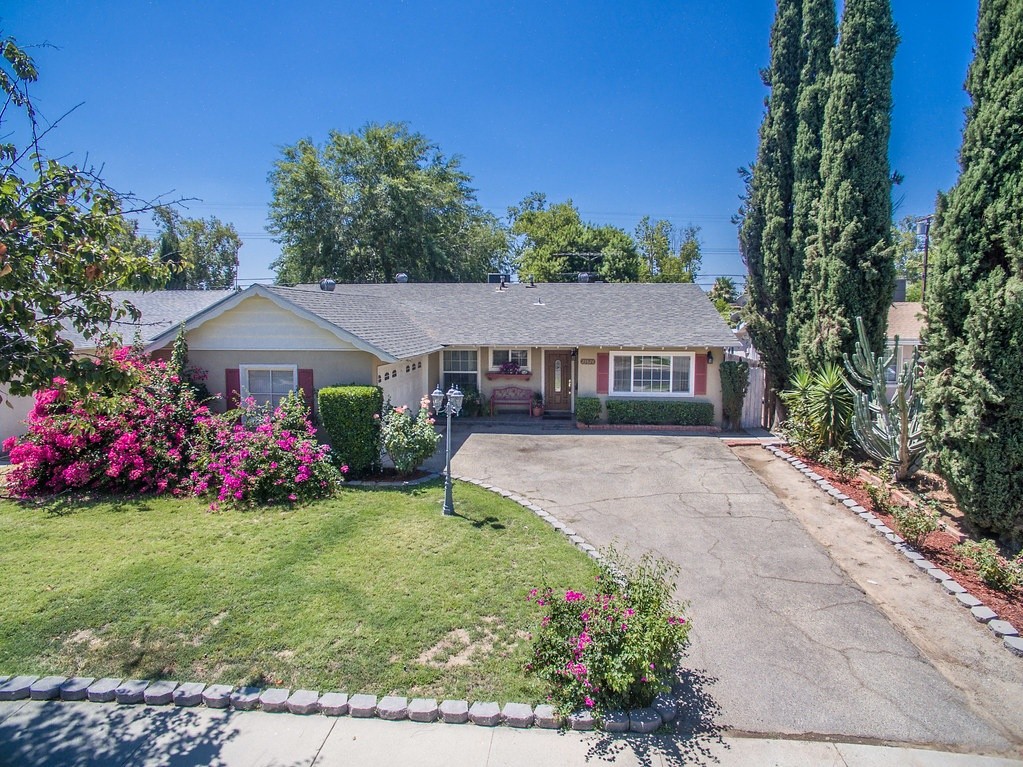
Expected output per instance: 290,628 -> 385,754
571,351 -> 576,361
707,351 -> 713,364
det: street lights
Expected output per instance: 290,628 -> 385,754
431,383 -> 465,514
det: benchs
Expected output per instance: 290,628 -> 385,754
490,387 -> 533,417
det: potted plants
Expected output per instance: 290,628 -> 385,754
461,387 -> 488,417
530,391 -> 545,417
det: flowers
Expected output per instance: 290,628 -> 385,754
500,362 -> 520,374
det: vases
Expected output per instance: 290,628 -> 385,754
503,369 -> 514,375
